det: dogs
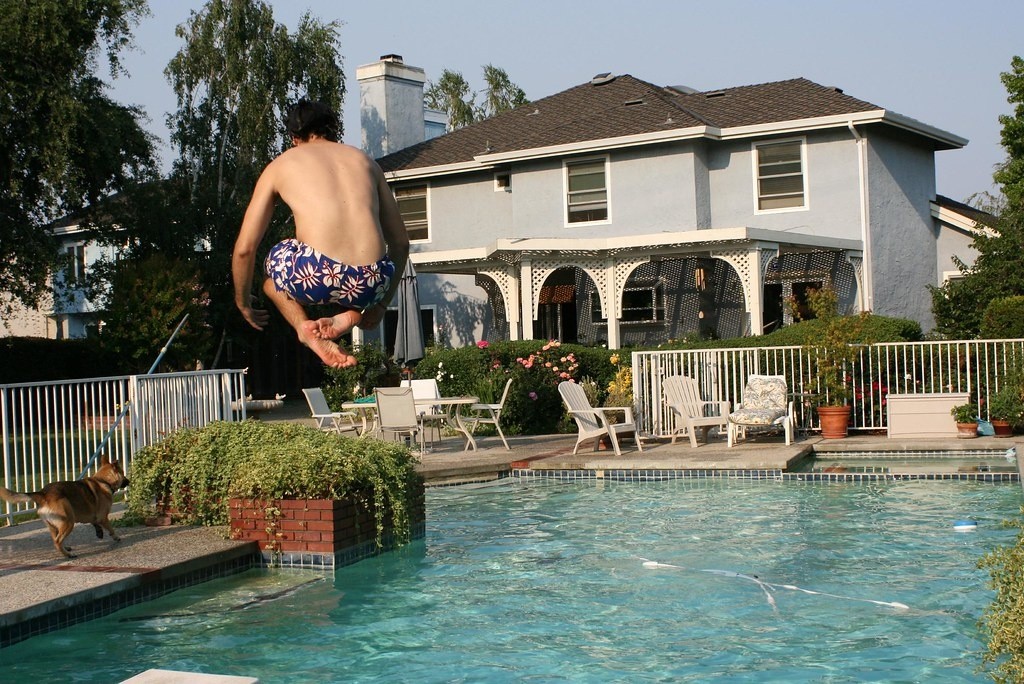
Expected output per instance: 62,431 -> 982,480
0,454 -> 131,558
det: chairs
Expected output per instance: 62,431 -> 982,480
373,386 -> 427,458
727,374 -> 795,446
465,378 -> 513,451
400,379 -> 443,449
661,375 -> 732,447
558,382 -> 642,456
303,388 -> 363,435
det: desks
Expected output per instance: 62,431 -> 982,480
342,395 -> 480,452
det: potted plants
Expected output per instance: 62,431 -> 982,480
802,284 -> 876,440
987,385 -> 1024,438
950,404 -> 979,438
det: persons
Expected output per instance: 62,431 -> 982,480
230,99 -> 410,370
